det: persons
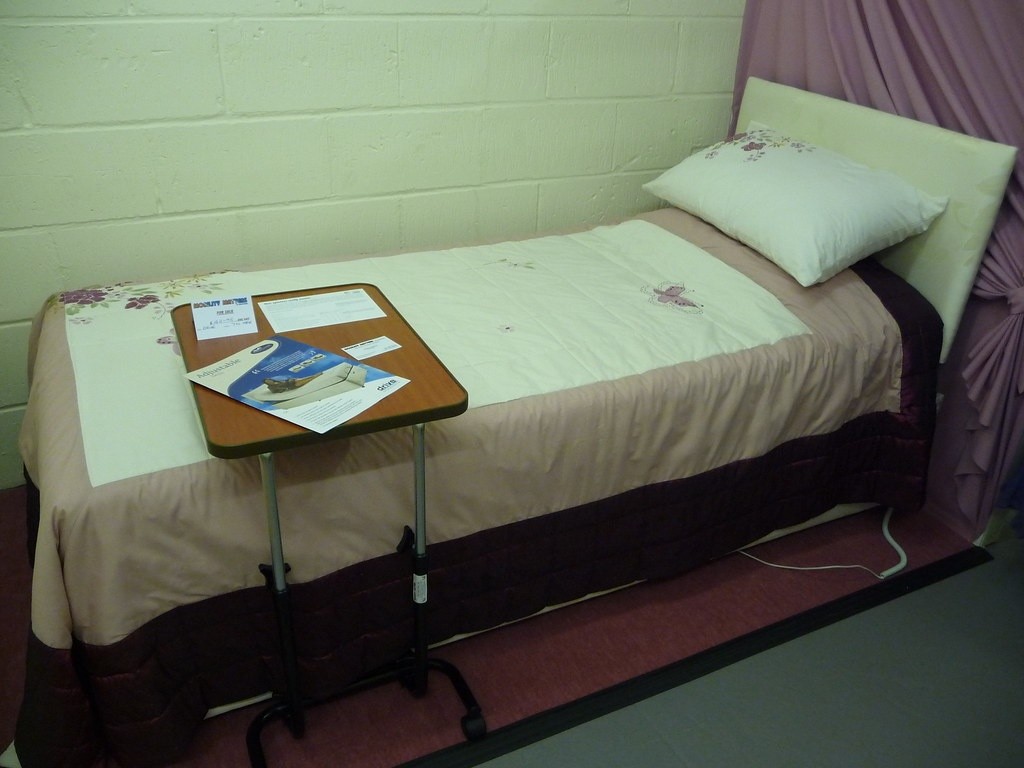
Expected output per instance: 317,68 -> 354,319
263,372 -> 323,393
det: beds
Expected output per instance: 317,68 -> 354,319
14,75 -> 1018,768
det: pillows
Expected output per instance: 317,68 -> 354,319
640,118 -> 953,287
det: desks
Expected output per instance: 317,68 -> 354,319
168,282 -> 490,768
